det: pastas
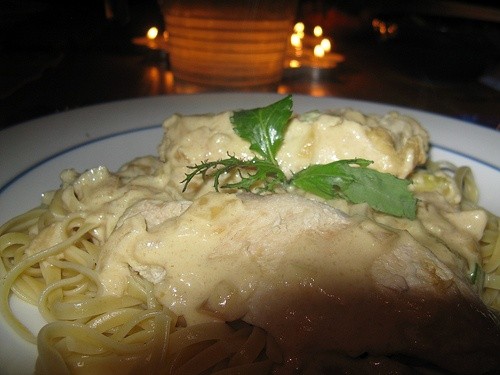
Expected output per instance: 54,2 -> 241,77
0,95 -> 500,375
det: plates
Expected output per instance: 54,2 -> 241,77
0,92 -> 500,374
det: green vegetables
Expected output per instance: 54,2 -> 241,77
180,95 -> 417,219
